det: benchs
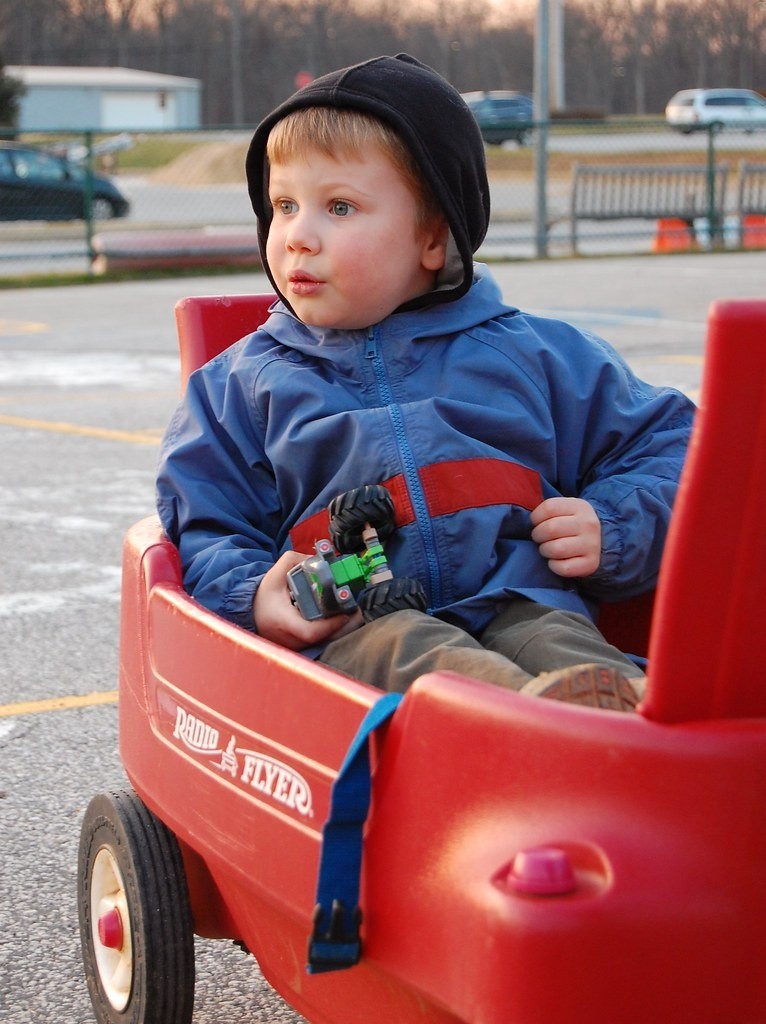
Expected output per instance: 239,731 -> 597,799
545,160 -> 766,258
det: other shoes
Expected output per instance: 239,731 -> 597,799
523,662 -> 638,715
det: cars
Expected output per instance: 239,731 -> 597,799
73,293 -> 766,1024
0,140 -> 130,224
665,87 -> 766,135
461,91 -> 531,145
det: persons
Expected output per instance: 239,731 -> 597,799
153,53 -> 698,712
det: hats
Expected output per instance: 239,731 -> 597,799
245,54 -> 491,319
